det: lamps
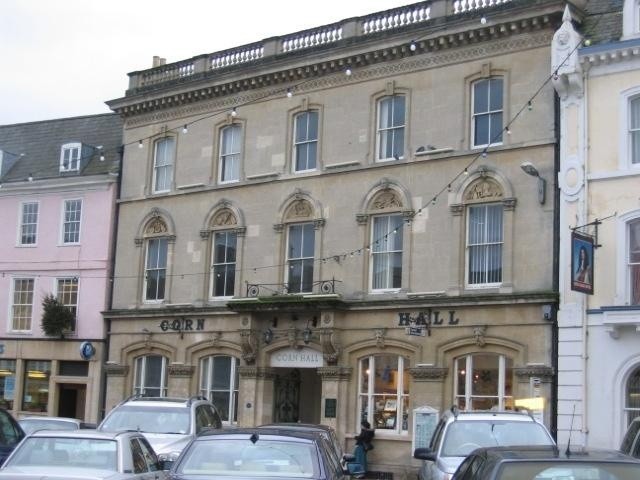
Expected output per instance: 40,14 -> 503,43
262,316 -> 278,344
301,316 -> 317,345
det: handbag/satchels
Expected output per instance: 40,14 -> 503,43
361,440 -> 373,451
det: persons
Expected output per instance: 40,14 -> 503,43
574,246 -> 593,288
354,421 -> 374,470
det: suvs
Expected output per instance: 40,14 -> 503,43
414,402 -> 558,479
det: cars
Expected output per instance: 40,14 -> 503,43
450,444 -> 640,479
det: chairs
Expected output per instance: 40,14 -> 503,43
202,460 -> 305,474
26,449 -> 53,464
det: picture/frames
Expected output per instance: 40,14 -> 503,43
571,232 -> 594,295
